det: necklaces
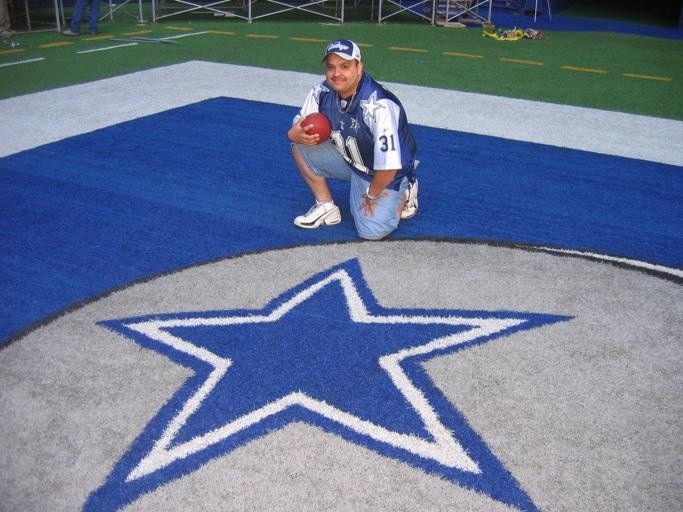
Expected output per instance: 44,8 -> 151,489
334,89 -> 357,115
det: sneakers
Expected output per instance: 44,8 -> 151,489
400,176 -> 419,219
293,200 -> 342,229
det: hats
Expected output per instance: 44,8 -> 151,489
321,38 -> 361,63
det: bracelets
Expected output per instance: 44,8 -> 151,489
365,187 -> 376,200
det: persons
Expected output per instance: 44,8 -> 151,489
287,39 -> 419,240
59,0 -> 99,36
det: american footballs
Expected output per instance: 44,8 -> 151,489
301,114 -> 330,144
